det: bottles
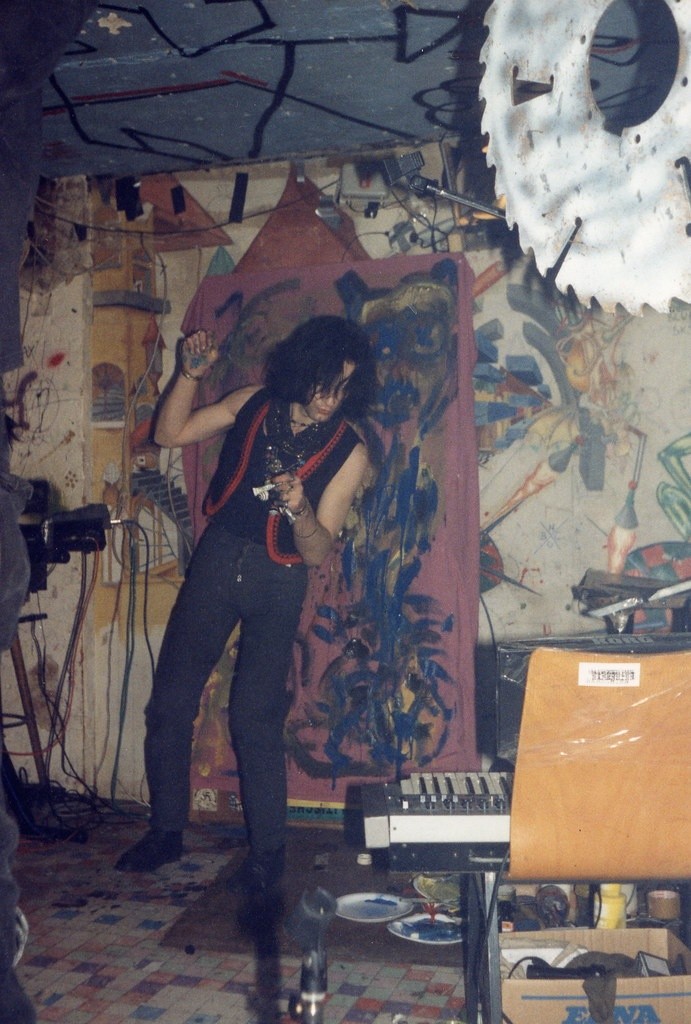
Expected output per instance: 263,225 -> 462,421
594,884 -> 628,929
498,901 -> 518,933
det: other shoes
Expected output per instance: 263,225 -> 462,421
118,831 -> 184,871
226,859 -> 271,893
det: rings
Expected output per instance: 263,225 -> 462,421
284,473 -> 295,494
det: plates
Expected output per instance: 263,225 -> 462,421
386,913 -> 463,946
334,892 -> 414,924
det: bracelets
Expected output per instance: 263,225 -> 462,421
180,367 -> 204,381
292,519 -> 319,538
292,498 -> 309,518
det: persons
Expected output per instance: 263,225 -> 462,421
111,316 -> 377,895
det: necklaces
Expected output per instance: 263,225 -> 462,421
263,398 -> 328,483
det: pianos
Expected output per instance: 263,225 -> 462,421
356,770 -> 514,871
